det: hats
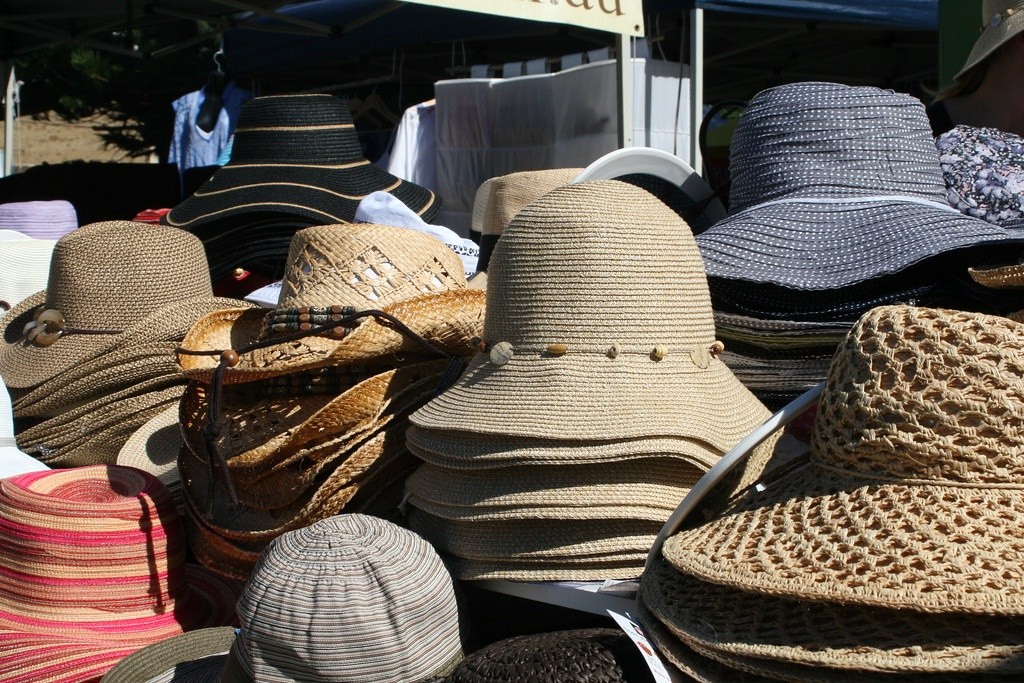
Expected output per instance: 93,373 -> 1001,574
99,513 -> 480,683
0,464 -> 248,683
158,95 -> 444,280
693,81 -> 1024,411
404,179 -> 812,583
443,628 -> 657,683
634,304 -> 1024,683
952,0 -> 1024,80
176,222 -> 487,582
115,398 -> 186,516
243,190 -> 481,309
469,145 -> 728,272
0,199 -> 263,469
